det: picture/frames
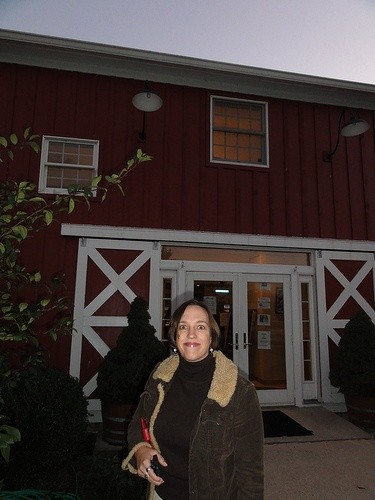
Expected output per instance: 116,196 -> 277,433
275,287 -> 284,314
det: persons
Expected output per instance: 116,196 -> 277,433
122,299 -> 266,500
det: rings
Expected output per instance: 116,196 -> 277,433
146,465 -> 152,472
144,473 -> 150,478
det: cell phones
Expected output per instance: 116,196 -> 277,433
153,454 -> 160,476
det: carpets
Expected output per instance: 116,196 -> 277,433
262,410 -> 315,437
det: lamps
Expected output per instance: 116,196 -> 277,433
323,107 -> 370,162
131,80 -> 164,143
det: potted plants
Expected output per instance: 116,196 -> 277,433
329,311 -> 375,427
96,296 -> 170,446
